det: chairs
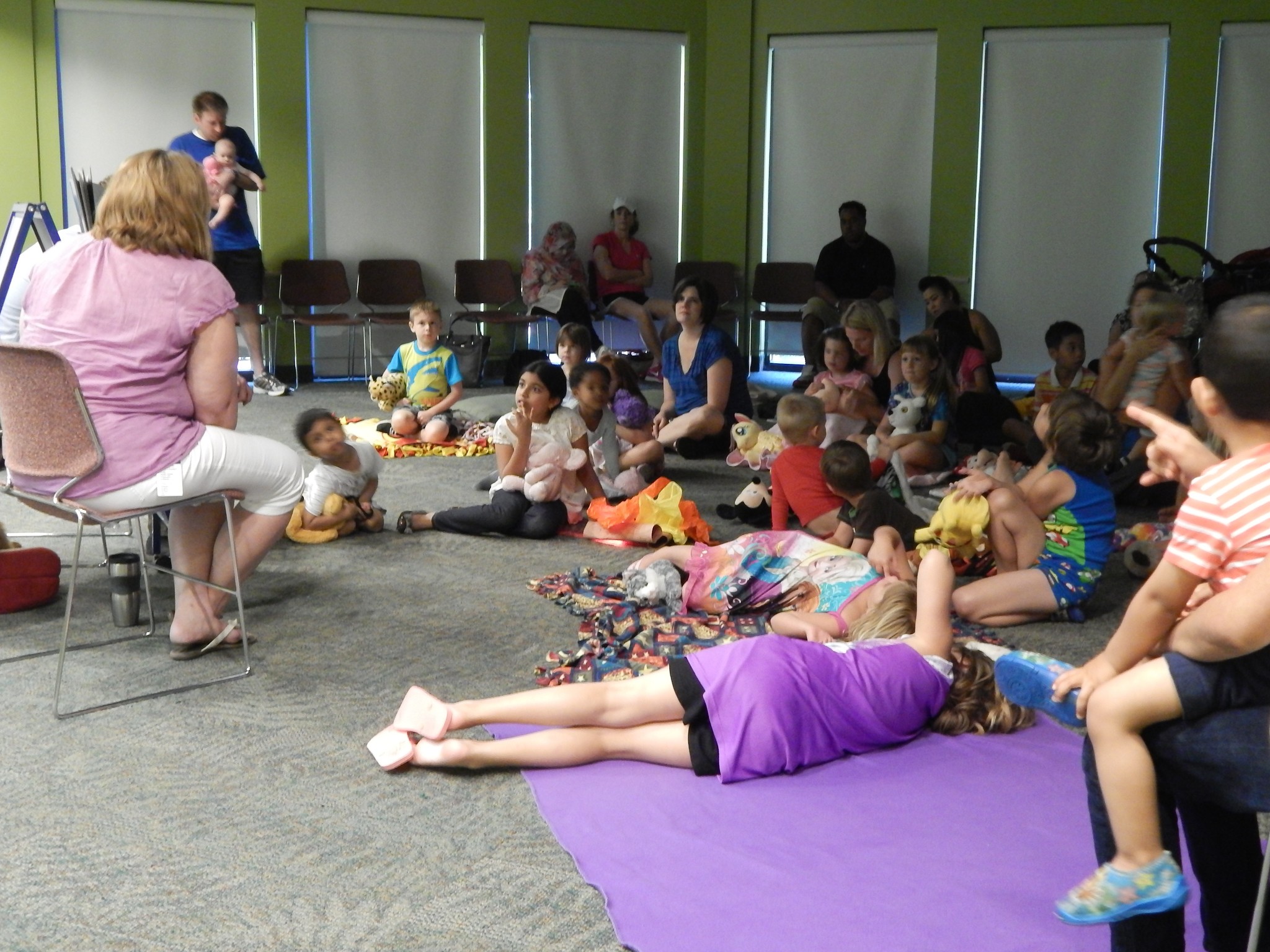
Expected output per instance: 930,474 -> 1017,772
350,259 -> 442,382
272,258 -> 368,387
746,261 -> 815,378
585,257 -> 663,352
447,257 -> 543,387
233,271 -> 274,376
517,257 -> 607,354
671,260 -> 741,347
0,337 -> 256,722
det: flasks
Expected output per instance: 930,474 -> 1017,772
108,552 -> 141,628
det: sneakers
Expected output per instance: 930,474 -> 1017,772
253,372 -> 290,397
645,364 -> 664,384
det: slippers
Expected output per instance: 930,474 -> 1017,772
398,510 -> 426,533
169,619 -> 258,661
366,726 -> 413,770
393,686 -> 452,741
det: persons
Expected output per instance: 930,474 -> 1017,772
650,277 -> 753,459
0,225 -> 169,537
626,523 -> 925,644
591,199 -> 680,384
293,408 -> 385,538
770,200 -> 1270,951
396,321 -> 664,539
522,220 -> 620,359
376,300 -> 463,442
200,139 -> 267,230
10,148 -> 304,662
367,546 -> 1036,783
168,92 -> 288,396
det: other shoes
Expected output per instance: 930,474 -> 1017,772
792,364 -> 819,387
1051,848 -> 1189,925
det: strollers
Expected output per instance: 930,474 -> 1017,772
1143,235 -> 1270,325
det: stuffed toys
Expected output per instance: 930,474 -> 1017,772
731,413 -> 785,464
716,477 -> 796,526
912,490 -> 991,564
807,378 -> 868,419
368,371 -> 407,412
502,437 -> 588,503
867,393 -> 926,460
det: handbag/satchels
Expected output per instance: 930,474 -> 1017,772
994,651 -> 1088,728
437,314 -> 490,387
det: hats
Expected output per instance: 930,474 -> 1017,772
613,197 -> 637,214
